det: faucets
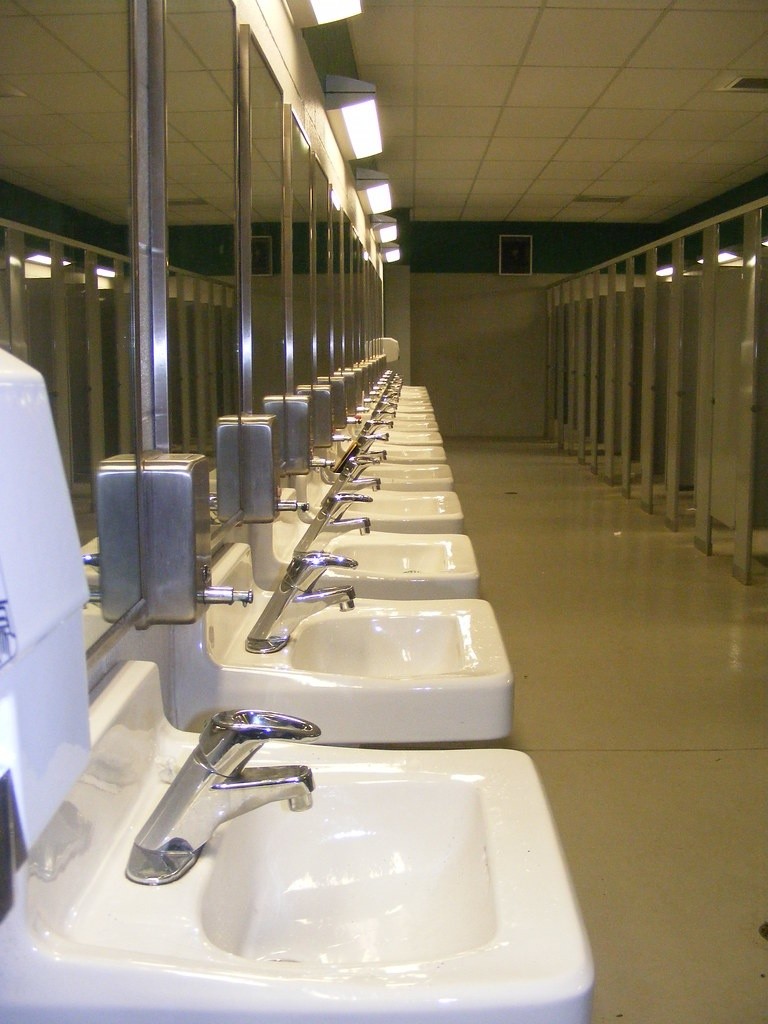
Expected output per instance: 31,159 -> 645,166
125,707 -> 323,886
361,420 -> 394,434
334,432 -> 390,473
82,553 -> 100,603
244,549 -> 360,654
375,373 -> 403,420
292,493 -> 374,552
329,456 -> 382,492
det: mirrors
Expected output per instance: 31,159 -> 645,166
0,0 -> 388,673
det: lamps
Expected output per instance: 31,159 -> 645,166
286,0 -> 363,30
696,249 -> 739,265
655,264 -> 673,277
369,214 -> 398,244
356,167 -> 393,216
96,266 -> 116,279
379,242 -> 400,264
325,73 -> 383,161
24,253 -> 73,280
761,239 -> 768,247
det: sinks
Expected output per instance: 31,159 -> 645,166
357,461 -> 454,491
393,385 -> 439,431
368,442 -> 447,464
327,485 -> 464,534
272,593 -> 514,744
374,430 -> 444,447
158,737 -> 595,1024
304,528 -> 480,599
